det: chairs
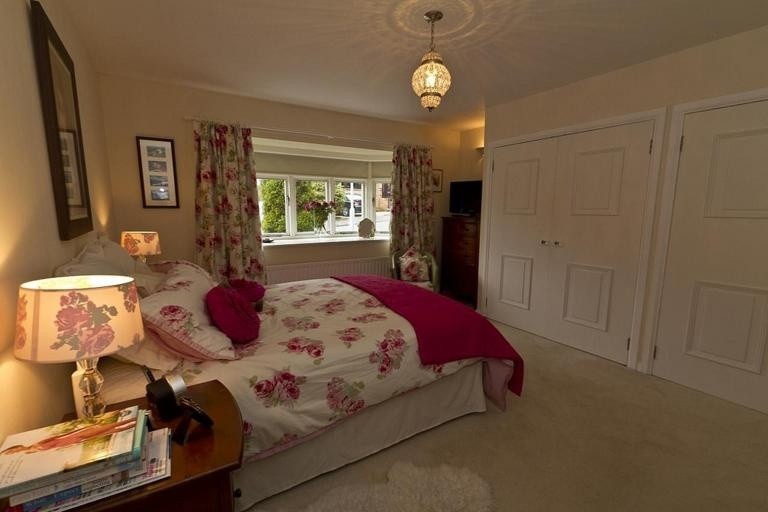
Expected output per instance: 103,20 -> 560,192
391,247 -> 438,292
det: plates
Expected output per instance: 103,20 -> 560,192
262,238 -> 273,243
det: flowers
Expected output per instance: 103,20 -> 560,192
302,199 -> 338,231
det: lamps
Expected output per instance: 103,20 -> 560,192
14,273 -> 146,416
410,9 -> 453,114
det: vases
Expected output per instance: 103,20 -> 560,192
313,211 -> 327,238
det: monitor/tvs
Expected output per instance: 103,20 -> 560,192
449,180 -> 482,217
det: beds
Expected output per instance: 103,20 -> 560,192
96,275 -> 515,512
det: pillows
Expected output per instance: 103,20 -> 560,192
400,246 -> 429,281
51,231 -> 267,371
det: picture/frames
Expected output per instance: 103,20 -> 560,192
58,127 -> 85,210
33,1 -> 95,241
432,168 -> 443,193
136,135 -> 179,208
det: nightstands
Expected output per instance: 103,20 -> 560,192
2,378 -> 246,512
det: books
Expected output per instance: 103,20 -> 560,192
0,405 -> 173,512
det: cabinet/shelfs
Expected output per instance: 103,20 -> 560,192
440,215 -> 480,306
